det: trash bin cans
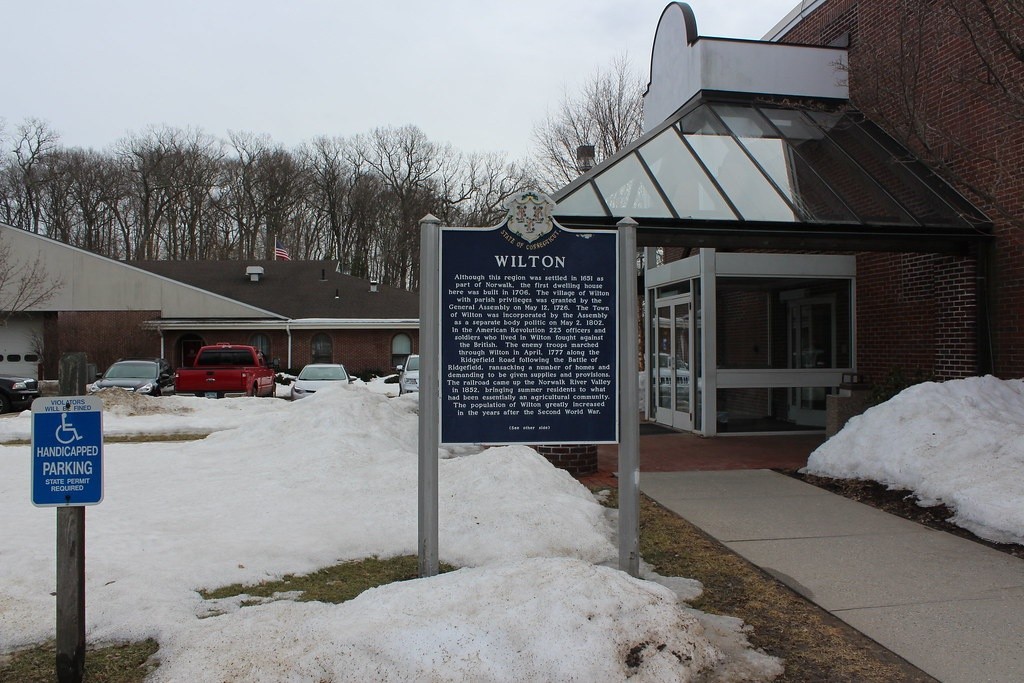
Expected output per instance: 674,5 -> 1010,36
838,371 -> 874,399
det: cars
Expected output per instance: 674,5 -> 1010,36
0,371 -> 41,415
395,354 -> 420,397
290,363 -> 357,403
651,352 -> 696,397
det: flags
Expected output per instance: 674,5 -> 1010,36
274,237 -> 290,259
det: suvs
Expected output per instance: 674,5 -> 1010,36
88,356 -> 176,399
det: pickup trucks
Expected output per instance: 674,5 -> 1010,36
173,342 -> 278,401
